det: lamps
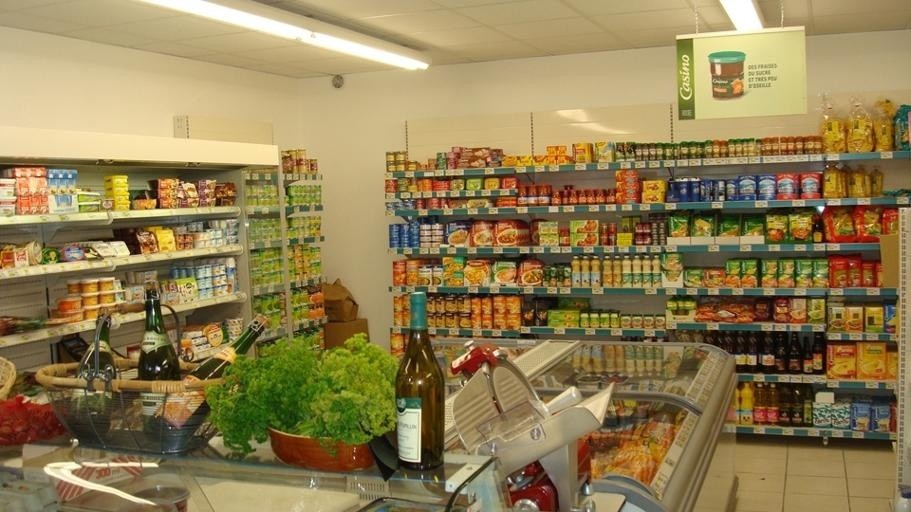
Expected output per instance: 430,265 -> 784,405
148,0 -> 435,73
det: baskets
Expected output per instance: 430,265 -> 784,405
35,359 -> 245,457
0,355 -> 17,401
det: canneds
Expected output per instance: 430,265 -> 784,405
580,309 -> 620,328
385,150 -> 406,171
394,295 -> 523,329
615,135 -> 824,161
389,223 -> 445,248
281,149 -> 318,174
654,314 -> 665,328
393,259 -> 444,286
708,51 -> 746,98
408,160 -> 417,171
620,314 -> 632,328
515,185 -> 617,206
599,223 -> 617,246
631,314 -> 642,328
385,178 -> 449,210
643,314 -> 654,329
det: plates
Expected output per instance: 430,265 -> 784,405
447,227 -> 545,285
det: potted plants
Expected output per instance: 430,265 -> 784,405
203,332 -> 402,473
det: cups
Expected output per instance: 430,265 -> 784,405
131,484 -> 191,511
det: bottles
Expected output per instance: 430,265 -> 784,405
572,344 -> 664,378
737,382 -> 815,424
678,329 -> 826,373
617,135 -> 825,160
572,254 -> 661,287
149,314 -> 272,449
396,290 -> 445,470
580,308 -> 667,329
138,279 -> 180,442
66,306 -> 114,439
667,295 -> 697,314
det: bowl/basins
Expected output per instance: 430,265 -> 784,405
268,423 -> 376,471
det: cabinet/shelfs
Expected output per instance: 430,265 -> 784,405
383,147 -> 911,447
0,128 -> 281,406
246,170 -> 328,342
